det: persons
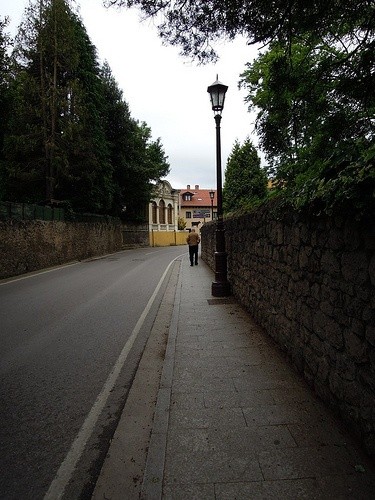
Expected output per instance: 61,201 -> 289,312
186,229 -> 200,266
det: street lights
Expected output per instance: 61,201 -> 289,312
209,190 -> 215,220
206,74 -> 237,305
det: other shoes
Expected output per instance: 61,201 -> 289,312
195,263 -> 199,265
190,264 -> 193,266
